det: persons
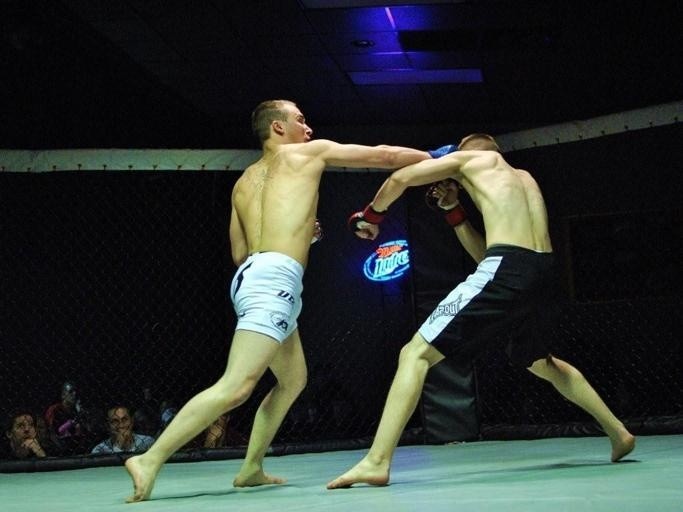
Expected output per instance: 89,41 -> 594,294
0,381 -> 247,461
325,134 -> 636,489
125,99 -> 458,503
